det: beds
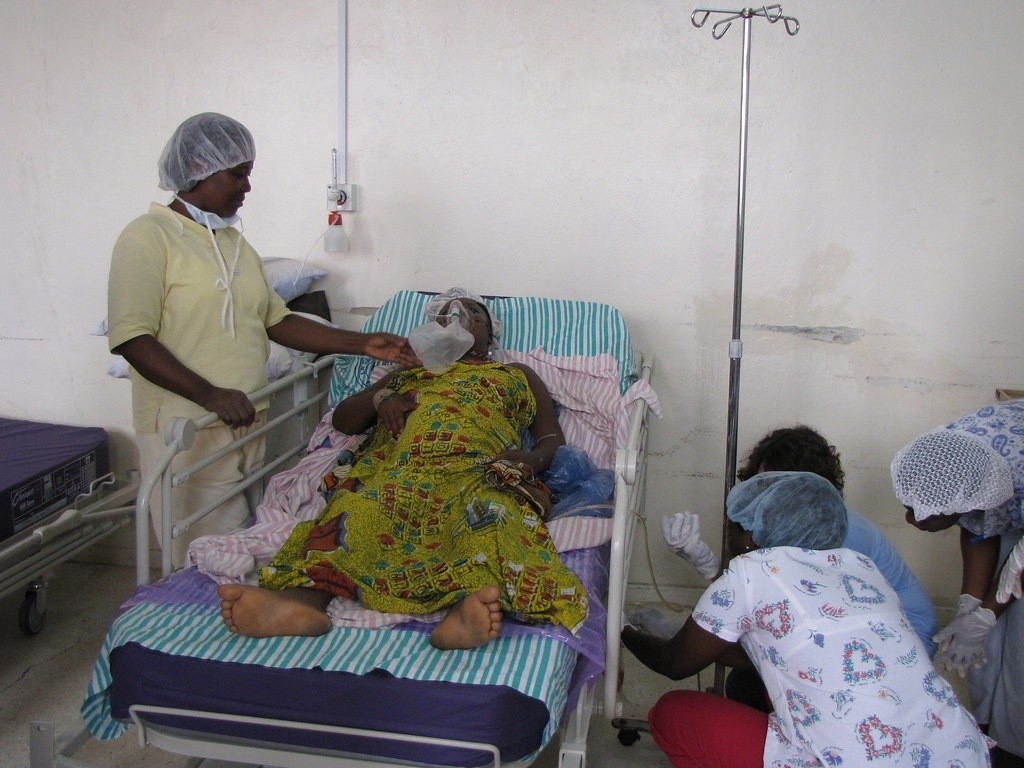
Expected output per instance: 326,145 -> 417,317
0,418 -> 142,637
29,293 -> 663,768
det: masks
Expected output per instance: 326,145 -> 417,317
176,194 -> 241,230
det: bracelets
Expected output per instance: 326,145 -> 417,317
373,389 -> 396,410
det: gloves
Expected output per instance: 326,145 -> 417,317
661,512 -> 720,580
996,535 -> 1024,604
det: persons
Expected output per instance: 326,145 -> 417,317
216,298 -> 567,649
891,399 -> 1024,768
107,112 -> 423,571
619,470 -> 997,768
661,425 -> 938,716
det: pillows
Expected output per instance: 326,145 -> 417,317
285,290 -> 332,323
89,258 -> 330,337
108,311 -> 339,383
